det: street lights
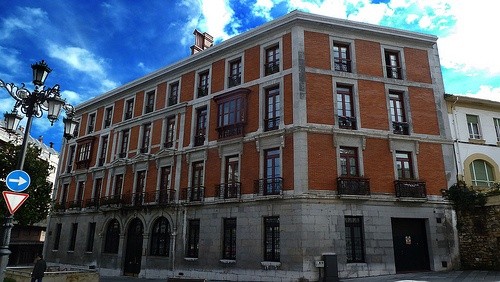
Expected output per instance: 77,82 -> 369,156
0,60 -> 68,282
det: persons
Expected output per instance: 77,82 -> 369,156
30,253 -> 47,282
